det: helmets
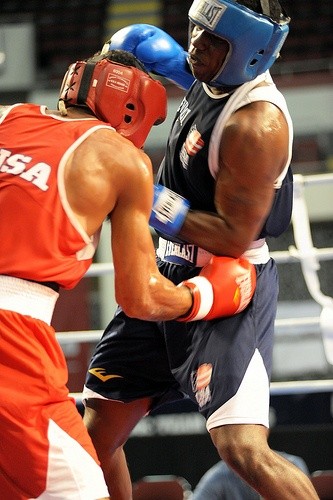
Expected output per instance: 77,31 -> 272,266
58,56 -> 168,149
188,0 -> 291,87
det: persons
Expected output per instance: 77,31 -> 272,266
98,0 -> 324,500
0,48 -> 258,500
190,404 -> 314,500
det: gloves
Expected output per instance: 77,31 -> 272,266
177,256 -> 257,322
148,183 -> 190,239
107,23 -> 194,90
